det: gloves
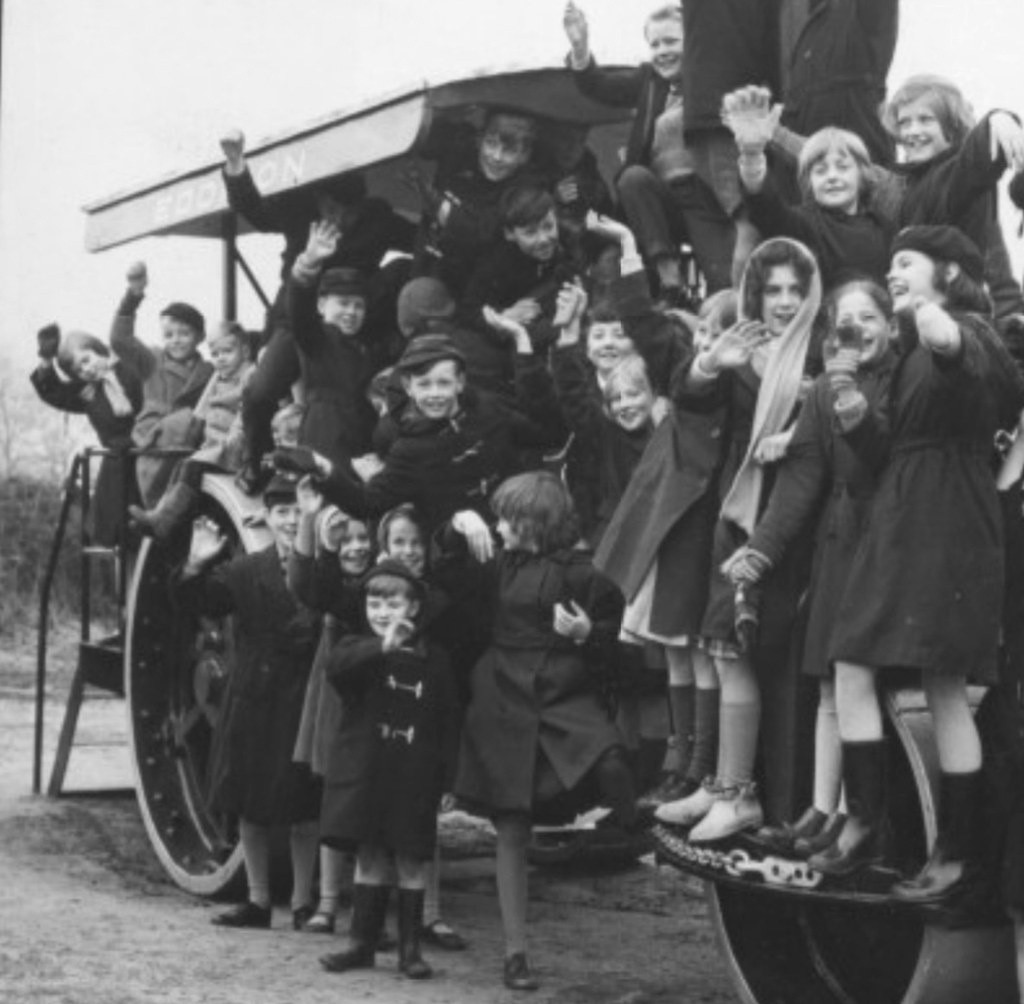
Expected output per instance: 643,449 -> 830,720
36,324 -> 60,360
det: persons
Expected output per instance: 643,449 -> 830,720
322,557 -> 460,978
431,474 -> 631,989
170,473 -> 320,927
30,1 -> 1024,949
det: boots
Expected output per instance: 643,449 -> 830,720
396,887 -> 433,980
125,482 -> 200,548
316,882 -> 391,970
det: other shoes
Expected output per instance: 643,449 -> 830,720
506,953 -> 536,990
424,918 -> 466,949
302,911 -> 335,934
374,931 -> 395,950
633,785 -> 987,905
293,906 -> 313,930
211,901 -> 271,929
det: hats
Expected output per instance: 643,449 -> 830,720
162,303 -> 205,335
320,267 -> 368,299
397,333 -> 466,370
262,475 -> 297,504
397,278 -> 456,327
358,559 -> 427,610
889,222 -> 985,287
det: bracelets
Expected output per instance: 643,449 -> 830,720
181,567 -> 200,575
